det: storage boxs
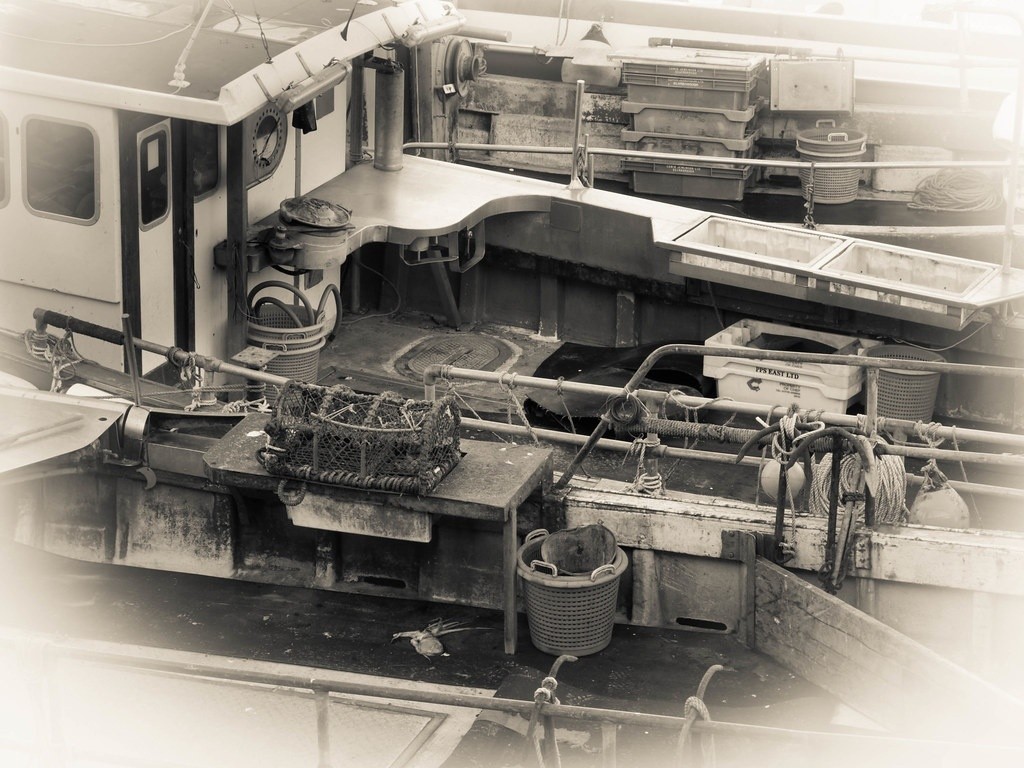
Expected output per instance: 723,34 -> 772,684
701,318 -> 883,415
872,145 -> 953,192
620,59 -> 766,201
